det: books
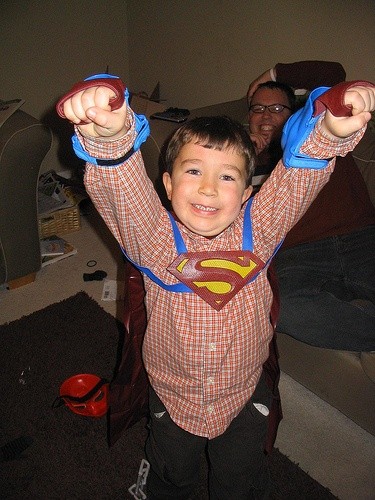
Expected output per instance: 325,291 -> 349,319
41,235 -> 78,268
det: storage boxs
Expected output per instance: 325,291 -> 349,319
38,185 -> 88,241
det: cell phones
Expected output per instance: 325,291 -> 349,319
152,113 -> 187,123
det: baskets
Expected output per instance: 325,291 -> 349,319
38,203 -> 80,240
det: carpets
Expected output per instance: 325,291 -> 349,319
0,291 -> 339,500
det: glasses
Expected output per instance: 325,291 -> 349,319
249,104 -> 291,113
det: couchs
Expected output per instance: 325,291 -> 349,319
140,95 -> 374,437
0,99 -> 54,291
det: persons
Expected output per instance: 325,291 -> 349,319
55,75 -> 375,496
250,61 -> 374,352
